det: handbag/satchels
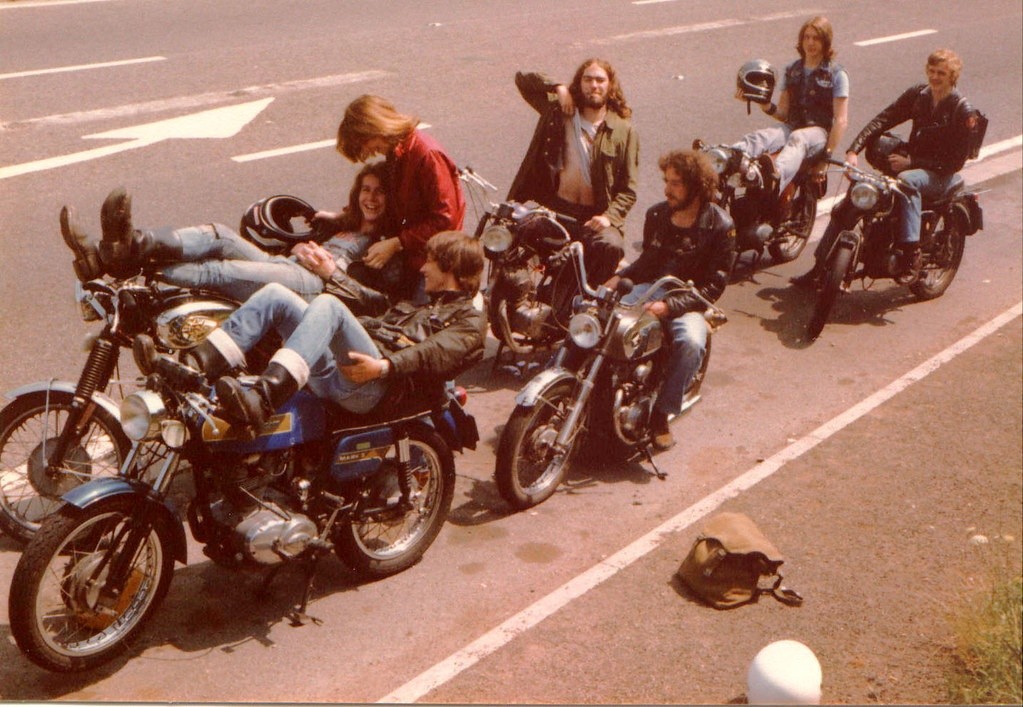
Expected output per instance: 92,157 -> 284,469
677,512 -> 804,609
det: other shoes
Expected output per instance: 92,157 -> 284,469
760,152 -> 779,193
693,137 -> 707,154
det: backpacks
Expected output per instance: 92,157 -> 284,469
911,83 -> 988,159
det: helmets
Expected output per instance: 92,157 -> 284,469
737,59 -> 775,104
239,195 -> 323,257
864,130 -> 909,176
747,639 -> 823,706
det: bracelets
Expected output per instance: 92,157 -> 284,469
379,357 -> 389,380
823,147 -> 833,155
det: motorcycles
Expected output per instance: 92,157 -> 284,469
0,137 -> 989,677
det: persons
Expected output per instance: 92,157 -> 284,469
788,49 -> 972,291
489,55 -> 638,294
337,96 -> 466,308
134,228 -> 488,444
57,159 -> 465,313
726,16 -> 851,200
598,147 -> 736,454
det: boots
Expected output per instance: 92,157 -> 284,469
216,348 -> 310,447
97,187 -> 183,272
60,205 -> 108,282
649,392 -> 674,451
790,256 -> 830,289
900,242 -> 922,284
133,327 -> 247,385
